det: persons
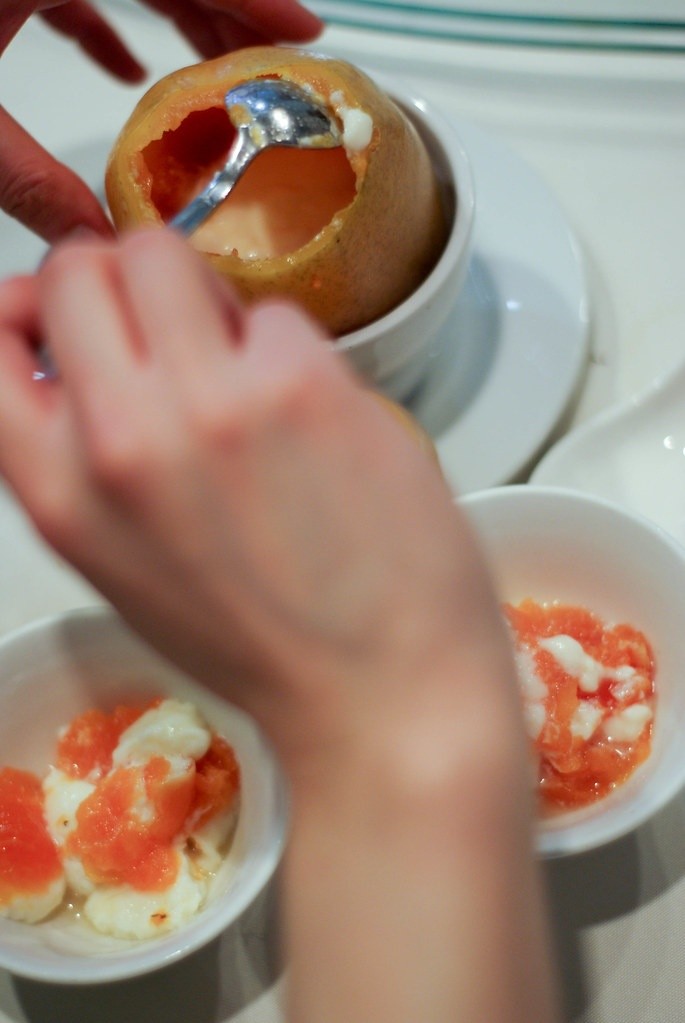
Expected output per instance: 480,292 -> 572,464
0,0 -> 552,1023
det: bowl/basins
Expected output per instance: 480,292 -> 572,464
331,58 -> 477,395
452,483 -> 685,861
0,607 -> 287,987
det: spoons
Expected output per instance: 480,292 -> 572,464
164,77 -> 347,240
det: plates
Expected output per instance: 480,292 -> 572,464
406,110 -> 592,494
